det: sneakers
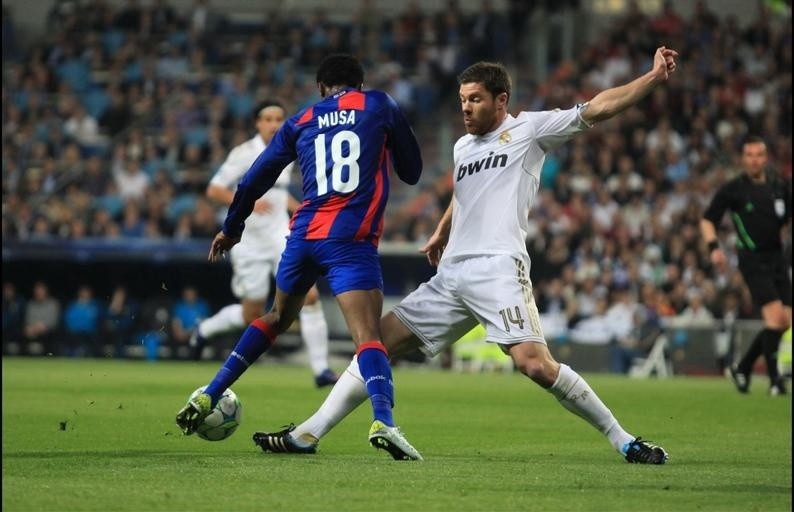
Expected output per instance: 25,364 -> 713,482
253,425 -> 316,455
369,420 -> 424,462
174,393 -> 214,436
621,439 -> 669,465
723,363 -> 752,395
768,381 -> 787,397
313,367 -> 341,389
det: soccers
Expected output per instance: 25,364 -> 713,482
186,385 -> 241,441
